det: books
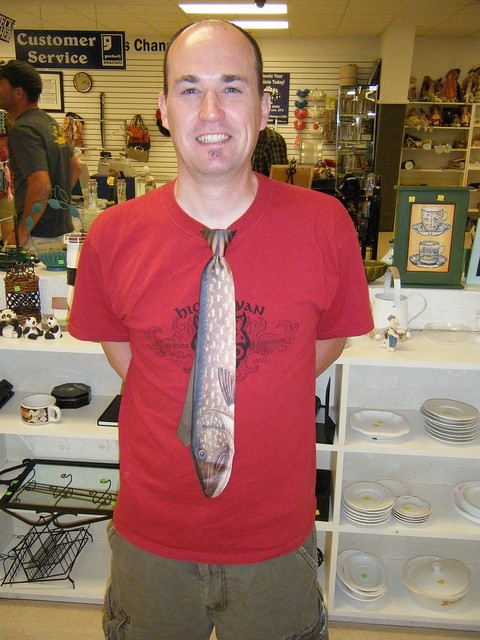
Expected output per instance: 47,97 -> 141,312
97,394 -> 123,427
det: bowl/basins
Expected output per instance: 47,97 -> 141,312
363,260 -> 387,282
336,573 -> 385,602
337,549 -> 388,597
401,555 -> 471,610
343,552 -> 388,591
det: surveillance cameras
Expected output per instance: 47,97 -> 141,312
254,0 -> 266,8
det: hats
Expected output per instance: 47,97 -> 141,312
1,59 -> 41,91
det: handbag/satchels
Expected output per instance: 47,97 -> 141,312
126,146 -> 149,162
128,115 -> 150,149
126,114 -> 144,139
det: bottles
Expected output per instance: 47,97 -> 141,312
135,178 -> 146,198
145,180 -> 155,193
116,179 -> 127,204
81,179 -> 100,232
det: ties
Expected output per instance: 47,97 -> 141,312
175,229 -> 238,498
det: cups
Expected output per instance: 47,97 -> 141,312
419,240 -> 445,264
421,209 -> 449,231
20,394 -> 62,426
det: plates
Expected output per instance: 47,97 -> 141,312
450,481 -> 480,525
350,409 -> 411,437
391,494 -> 431,525
459,483 -> 480,518
424,321 -> 475,347
376,479 -> 412,498
419,398 -> 480,444
342,481 -> 396,525
409,253 -> 447,268
412,222 -> 451,236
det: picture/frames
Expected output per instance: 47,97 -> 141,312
35,71 -> 64,113
392,184 -> 470,290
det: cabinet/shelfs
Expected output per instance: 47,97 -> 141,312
335,84 -> 379,201
393,102 -> 475,236
464,102 -> 480,215
0,328 -> 143,605
313,329 -> 480,632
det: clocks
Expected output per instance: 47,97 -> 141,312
72,72 -> 92,93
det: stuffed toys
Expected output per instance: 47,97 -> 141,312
0,309 -> 21,338
44,315 -> 62,340
22,317 -> 44,340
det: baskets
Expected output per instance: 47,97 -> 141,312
26,185 -> 83,268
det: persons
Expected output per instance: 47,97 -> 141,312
67,18 -> 374,640
251,126 -> 288,177
0,59 -> 83,256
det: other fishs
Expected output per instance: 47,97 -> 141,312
189,228 -> 239,499
